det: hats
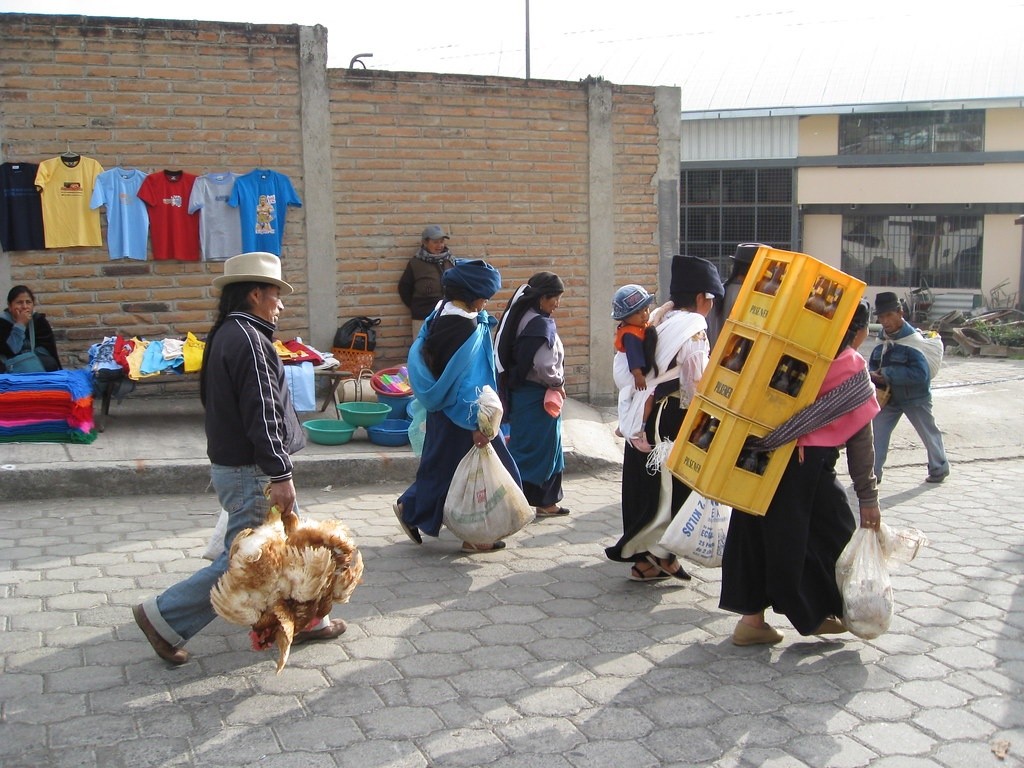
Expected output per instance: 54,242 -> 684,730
212,252 -> 294,296
729,243 -> 772,263
422,226 -> 451,240
872,292 -> 906,315
611,284 -> 655,321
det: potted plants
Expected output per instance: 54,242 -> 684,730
916,294 -> 932,310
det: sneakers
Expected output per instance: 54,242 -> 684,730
131,603 -> 187,663
292,618 -> 347,645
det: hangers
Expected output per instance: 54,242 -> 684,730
0,139 -> 278,180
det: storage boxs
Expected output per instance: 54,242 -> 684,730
663,247 -> 867,516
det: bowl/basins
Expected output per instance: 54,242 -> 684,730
406,398 -> 418,420
370,367 -> 414,397
366,418 -> 412,446
302,419 -> 357,445
336,401 -> 392,427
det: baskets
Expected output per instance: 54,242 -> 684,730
876,384 -> 891,410
329,333 -> 376,380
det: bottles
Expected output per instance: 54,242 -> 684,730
698,268 -> 842,472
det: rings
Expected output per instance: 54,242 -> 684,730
871,522 -> 876,525
866,520 -> 870,524
477,442 -> 481,445
28,313 -> 31,316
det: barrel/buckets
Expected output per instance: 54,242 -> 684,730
376,395 -> 414,420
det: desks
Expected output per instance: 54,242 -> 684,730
94,362 -> 353,431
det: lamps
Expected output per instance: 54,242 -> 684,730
964,203 -> 973,209
850,204 -> 861,210
905,204 -> 914,210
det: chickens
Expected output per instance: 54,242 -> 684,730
209,505 -> 366,676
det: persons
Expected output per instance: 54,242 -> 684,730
705,242 -> 882,644
869,292 -> 950,485
494,272 -> 570,516
611,284 -> 657,453
133,252 -> 347,664
604,254 -> 724,581
398,226 -> 458,345
393,258 -> 523,552
0,285 -> 63,374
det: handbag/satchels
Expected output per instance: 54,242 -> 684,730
4,351 -> 46,373
334,315 -> 381,351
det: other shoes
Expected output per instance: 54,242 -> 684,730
535,507 -> 570,516
731,620 -> 783,645
461,540 -> 506,552
645,554 -> 692,581
628,563 -> 672,580
925,472 -> 949,483
393,503 -> 423,545
810,616 -> 847,634
